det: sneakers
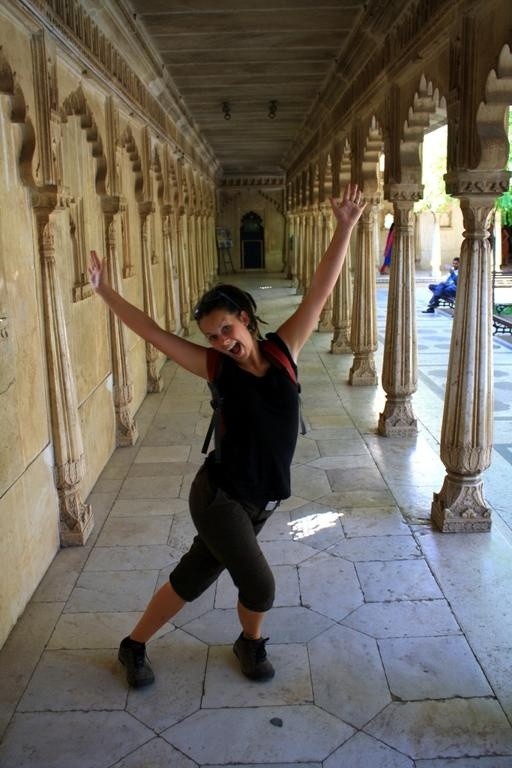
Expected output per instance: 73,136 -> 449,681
118,635 -> 155,689
232,630 -> 274,683
421,303 -> 438,313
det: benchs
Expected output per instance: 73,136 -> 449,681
493,303 -> 512,336
428,284 -> 456,308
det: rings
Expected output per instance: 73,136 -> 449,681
355,200 -> 360,206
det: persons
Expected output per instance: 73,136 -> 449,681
86,183 -> 367,687
422,258 -> 460,313
380,222 -> 394,275
